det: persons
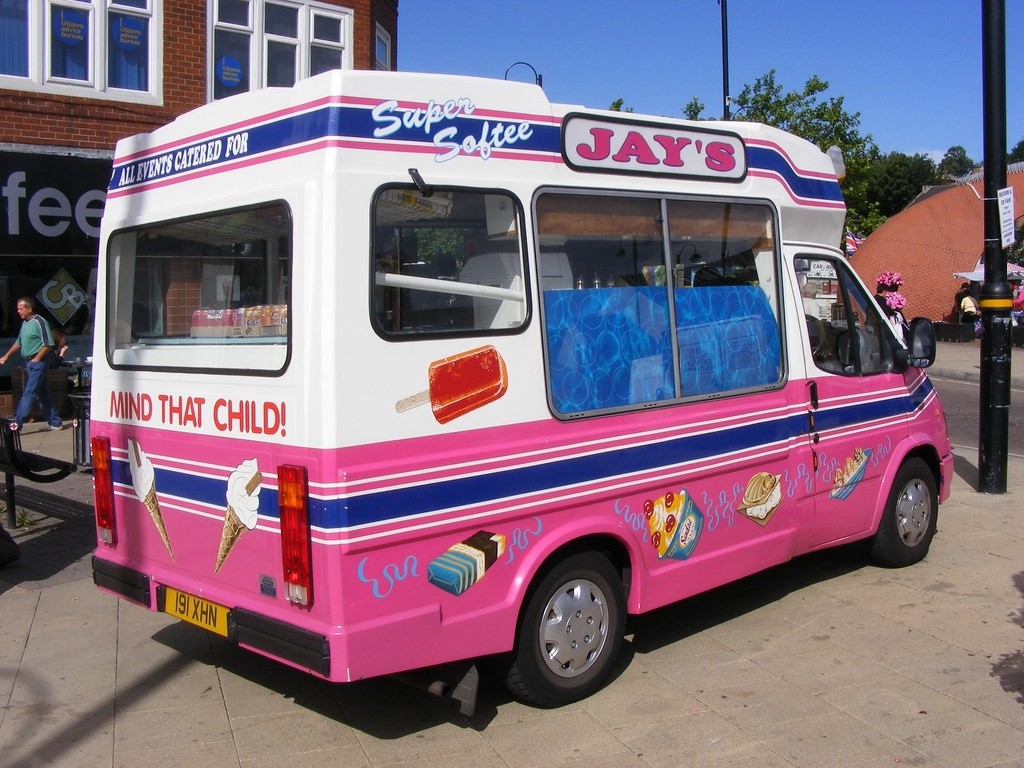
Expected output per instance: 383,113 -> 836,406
960,289 -> 980,325
48,327 -> 77,407
954,282 -> 971,324
1011,279 -> 1024,349
691,266 -> 723,287
874,283 -> 898,320
800,282 -> 823,321
973,308 -> 982,339
0,296 -> 64,436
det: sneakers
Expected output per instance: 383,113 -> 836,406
38,423 -> 63,431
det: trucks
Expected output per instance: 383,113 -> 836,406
87,68 -> 955,708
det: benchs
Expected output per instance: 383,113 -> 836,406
1,416 -> 75,527
